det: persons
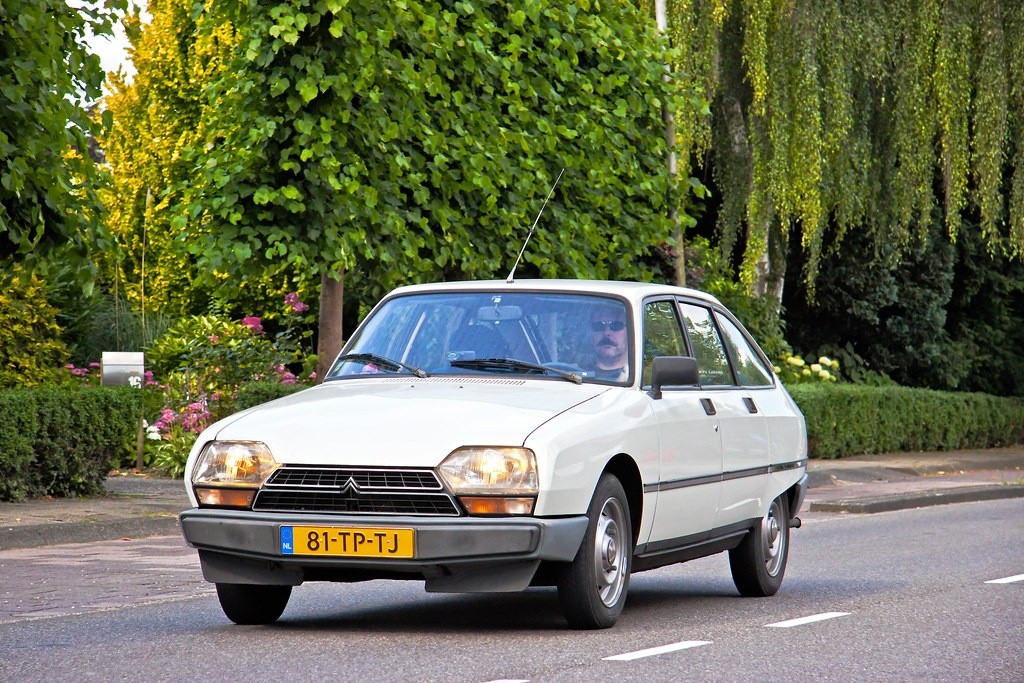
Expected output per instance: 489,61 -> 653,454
580,303 -> 629,382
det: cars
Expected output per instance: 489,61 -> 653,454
179,283 -> 808,623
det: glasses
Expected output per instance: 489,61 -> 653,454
591,320 -> 628,333
